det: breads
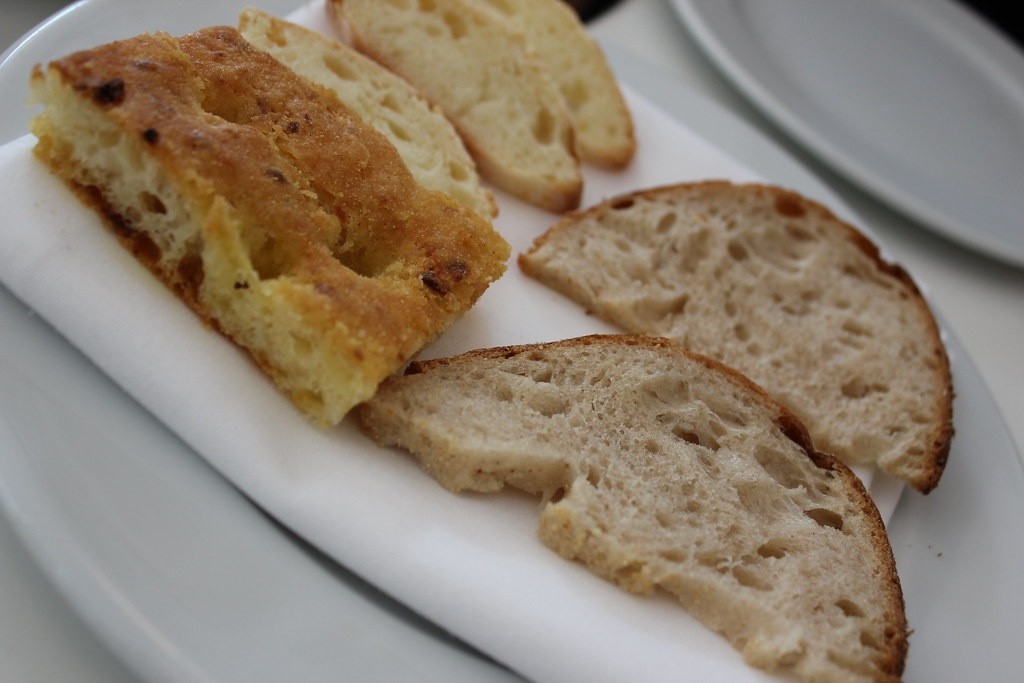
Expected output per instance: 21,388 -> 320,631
344,330 -> 908,683
239,9 -> 501,229
25,24 -> 512,426
323,0 -> 639,215
515,177 -> 956,497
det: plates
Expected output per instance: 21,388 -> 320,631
0,0 -> 1023,682
